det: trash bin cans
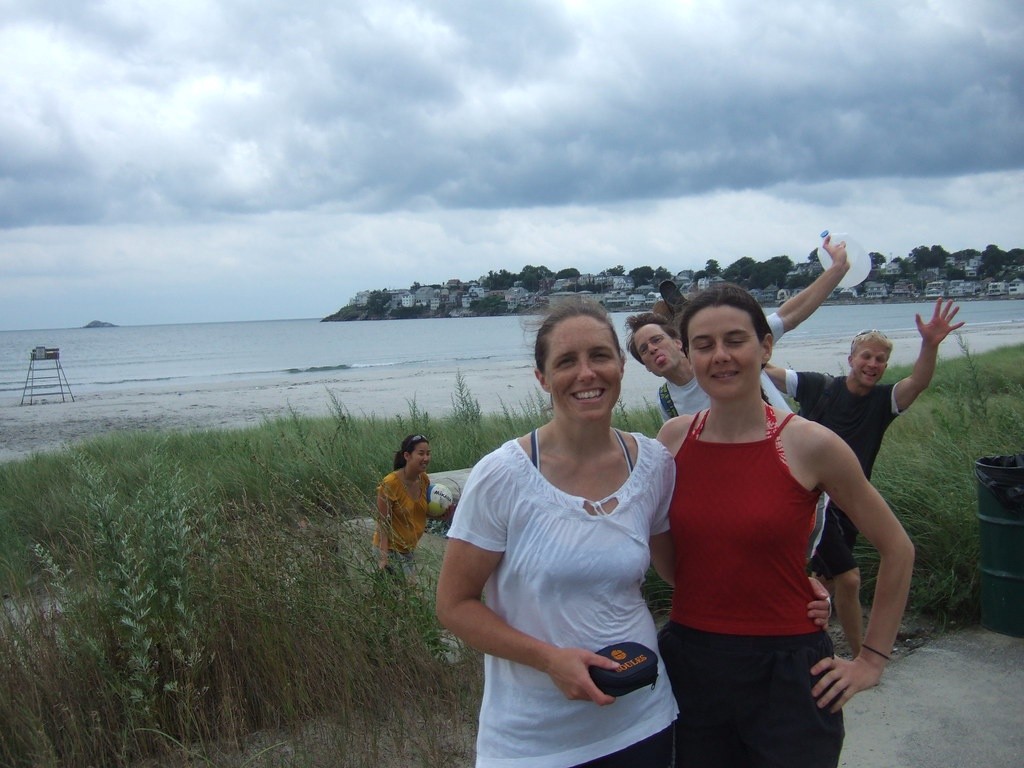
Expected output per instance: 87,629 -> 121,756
974,454 -> 1024,638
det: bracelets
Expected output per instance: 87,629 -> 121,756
862,644 -> 889,660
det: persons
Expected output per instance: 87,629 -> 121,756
624,235 -> 850,564
763,296 -> 965,658
656,285 -> 915,768
434,295 -> 831,768
371,432 -> 459,608
650,279 -> 693,334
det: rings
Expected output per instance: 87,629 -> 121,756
825,597 -> 830,603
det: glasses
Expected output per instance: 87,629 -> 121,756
406,435 -> 428,448
639,333 -> 669,356
853,329 -> 887,343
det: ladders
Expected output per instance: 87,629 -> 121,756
21,348 -> 74,405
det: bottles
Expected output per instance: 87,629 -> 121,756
817,231 -> 871,289
